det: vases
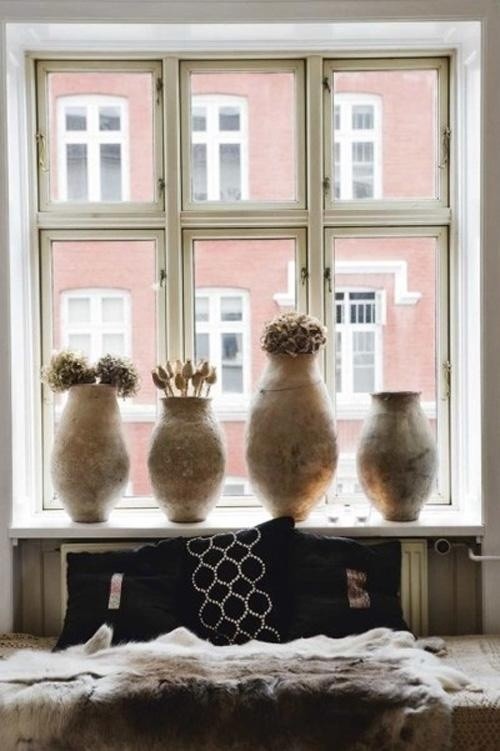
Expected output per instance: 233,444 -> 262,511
148,398 -> 224,522
244,353 -> 339,519
50,383 -> 131,522
356,390 -> 442,523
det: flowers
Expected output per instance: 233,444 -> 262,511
260,311 -> 327,356
40,348 -> 143,400
152,357 -> 217,397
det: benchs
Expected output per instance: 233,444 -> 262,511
0,633 -> 435,751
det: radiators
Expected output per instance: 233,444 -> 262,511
60,540 -> 429,636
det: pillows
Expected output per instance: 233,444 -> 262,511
53,516 -> 413,652
